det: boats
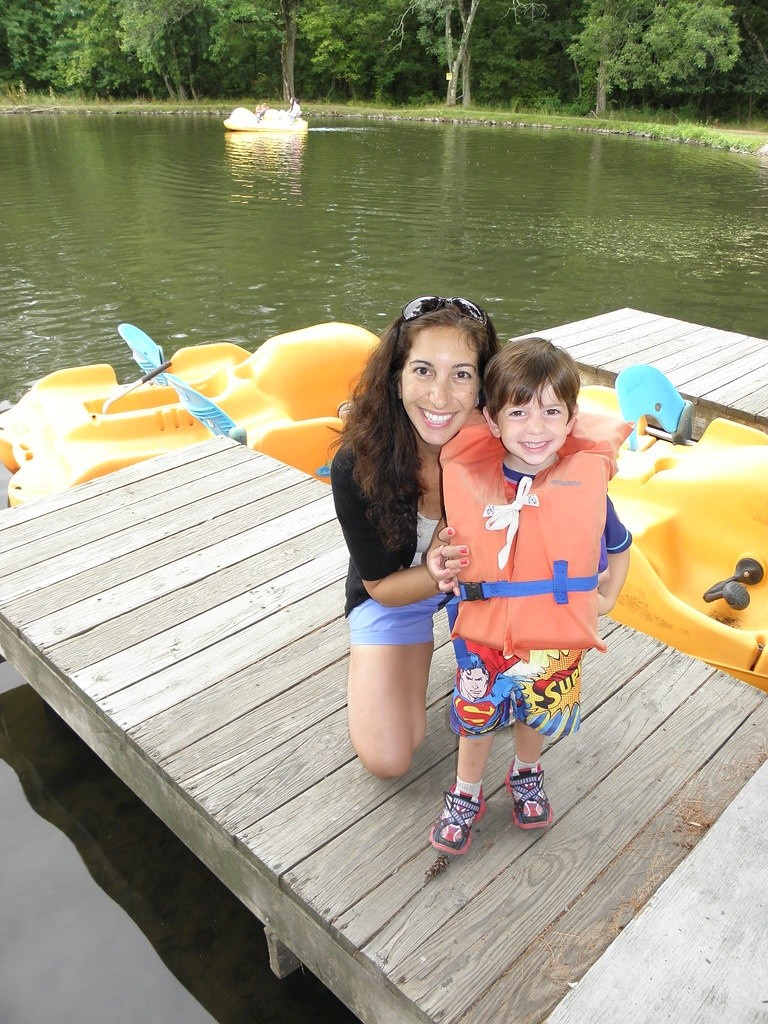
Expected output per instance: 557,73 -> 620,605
0,320 -> 390,511
570,362 -> 768,694
223,107 -> 309,133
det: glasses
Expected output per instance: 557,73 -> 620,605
389,295 -> 493,362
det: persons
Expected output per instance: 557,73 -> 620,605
428,338 -> 632,853
253,102 -> 271,121
329,295 -> 499,782
284,96 -> 302,123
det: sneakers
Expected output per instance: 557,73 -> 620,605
429,782 -> 486,856
504,759 -> 553,829
